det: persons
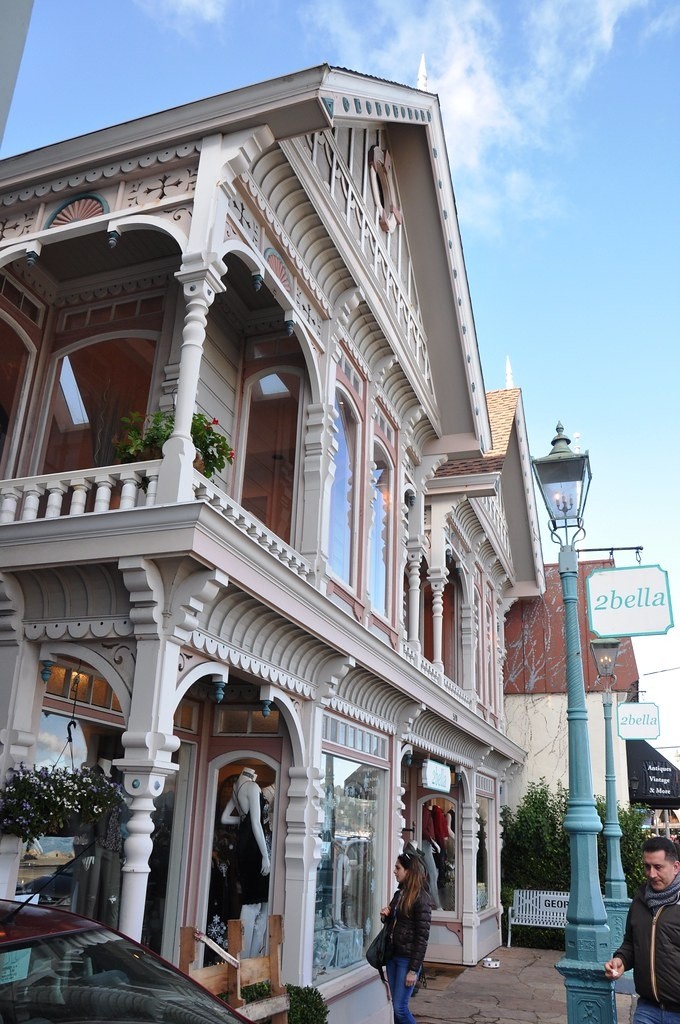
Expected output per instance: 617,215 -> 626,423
74,757 -> 130,931
604,837 -> 680,1024
380,853 -> 432,1024
422,830 -> 444,911
222,768 -> 272,956
317,843 -> 353,927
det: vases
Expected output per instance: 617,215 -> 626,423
44,812 -> 96,838
137,447 -> 203,471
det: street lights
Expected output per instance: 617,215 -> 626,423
588,637 -> 637,996
531,419 -> 617,1024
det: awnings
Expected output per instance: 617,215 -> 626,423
626,740 -> 680,810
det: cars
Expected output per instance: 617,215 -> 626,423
0,898 -> 258,1024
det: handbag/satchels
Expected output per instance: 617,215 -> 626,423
366,890 -> 400,1001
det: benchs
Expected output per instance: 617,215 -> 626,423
505,890 -> 570,951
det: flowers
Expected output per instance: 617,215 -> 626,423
1,761 -> 125,843
119,408 -> 236,494
